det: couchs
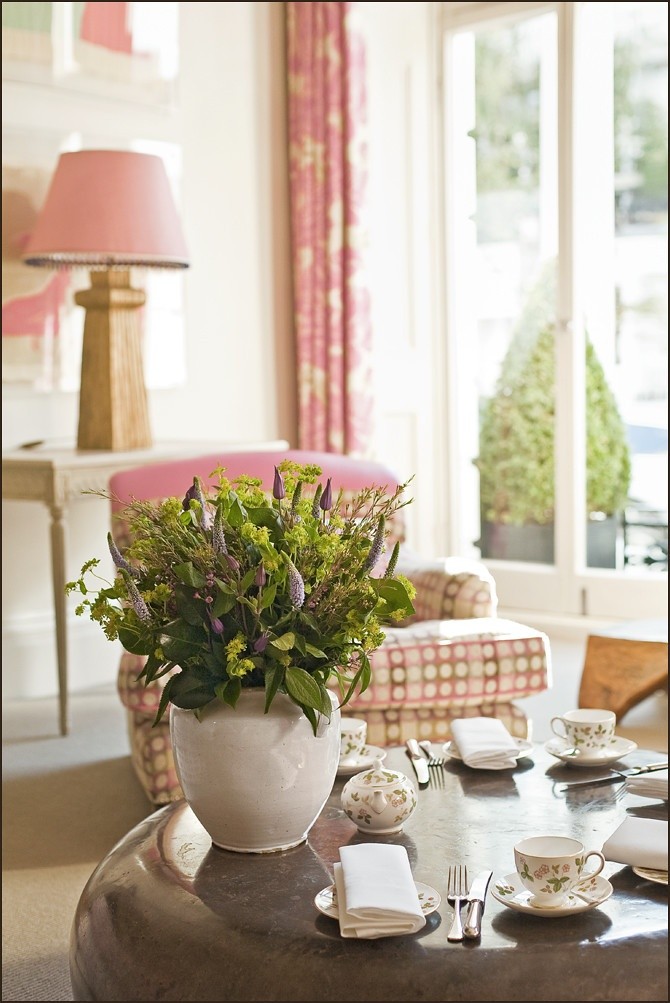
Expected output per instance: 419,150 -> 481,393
107,451 -> 551,809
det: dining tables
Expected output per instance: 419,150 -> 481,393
71,741 -> 668,1001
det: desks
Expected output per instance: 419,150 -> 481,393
3,433 -> 289,734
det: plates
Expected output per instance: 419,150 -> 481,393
336,745 -> 386,775
314,881 -> 441,920
443,737 -> 534,759
632,866 -> 668,885
545,735 -> 638,766
490,872 -> 613,917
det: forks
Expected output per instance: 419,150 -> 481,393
609,769 -> 628,779
447,865 -> 468,940
419,741 -> 445,766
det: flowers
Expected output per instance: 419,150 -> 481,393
62,458 -> 419,742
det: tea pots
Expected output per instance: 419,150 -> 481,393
341,759 -> 418,833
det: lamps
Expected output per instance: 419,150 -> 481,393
23,150 -> 190,449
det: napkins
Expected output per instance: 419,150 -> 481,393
601,816 -> 668,871
449,717 -> 518,769
333,843 -> 425,941
625,769 -> 668,800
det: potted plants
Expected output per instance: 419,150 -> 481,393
473,313 -> 632,568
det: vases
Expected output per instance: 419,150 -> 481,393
169,689 -> 343,853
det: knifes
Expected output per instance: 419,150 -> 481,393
462,870 -> 493,938
566,761 -> 668,785
406,739 -> 429,783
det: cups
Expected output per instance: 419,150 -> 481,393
550,709 -> 616,753
339,718 -> 367,766
514,836 -> 605,908
